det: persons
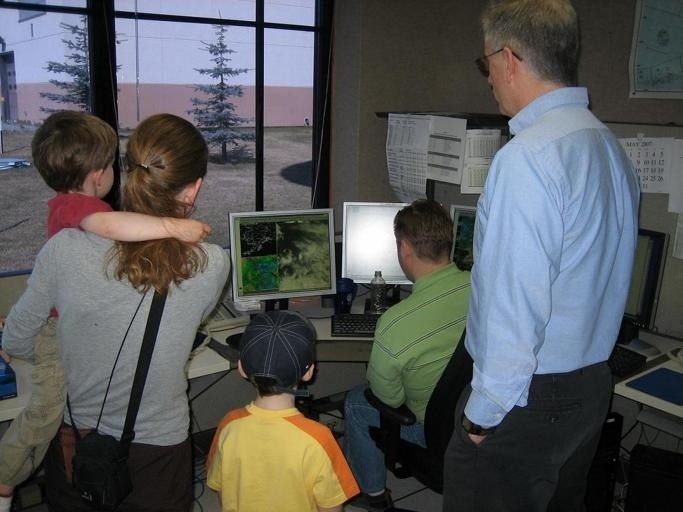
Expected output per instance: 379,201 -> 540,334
342,198 -> 471,512
441,0 -> 644,512
204,309 -> 361,512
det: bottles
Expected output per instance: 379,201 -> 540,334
370,271 -> 385,313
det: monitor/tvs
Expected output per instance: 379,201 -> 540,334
449,205 -> 477,272
226,207 -> 337,351
342,201 -> 414,310
617,228 -> 670,357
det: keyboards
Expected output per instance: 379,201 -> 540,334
609,345 -> 647,378
331,314 -> 382,337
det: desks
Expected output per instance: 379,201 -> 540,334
614,350 -> 683,456
0,284 -> 406,512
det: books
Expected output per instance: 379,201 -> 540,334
205,293 -> 250,331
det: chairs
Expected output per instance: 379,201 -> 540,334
364,319 -> 475,495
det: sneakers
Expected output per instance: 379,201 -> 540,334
346,489 -> 394,512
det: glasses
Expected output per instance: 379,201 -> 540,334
474,45 -> 525,78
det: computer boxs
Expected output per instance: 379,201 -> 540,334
584,412 -> 623,512
624,444 -> 683,512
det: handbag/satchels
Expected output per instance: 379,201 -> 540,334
68,430 -> 134,510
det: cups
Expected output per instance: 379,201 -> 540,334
333,277 -> 358,313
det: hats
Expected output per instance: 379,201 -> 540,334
239,310 -> 318,393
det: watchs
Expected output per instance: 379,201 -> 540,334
461,413 -> 495,437
